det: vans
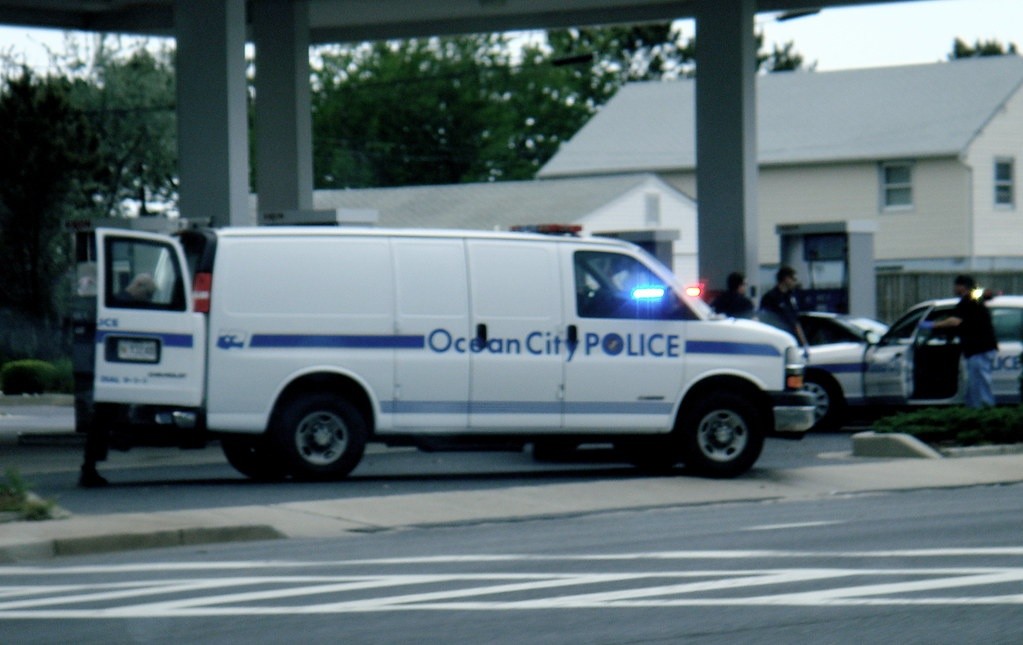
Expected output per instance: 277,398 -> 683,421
89,223 -> 804,487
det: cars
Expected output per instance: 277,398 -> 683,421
792,294 -> 1022,434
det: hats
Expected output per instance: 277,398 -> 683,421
955,275 -> 976,290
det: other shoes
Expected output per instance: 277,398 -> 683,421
78,465 -> 108,489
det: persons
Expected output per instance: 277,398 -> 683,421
713,272 -> 755,315
919,275 -> 999,410
759,266 -> 808,348
72,274 -> 155,487
